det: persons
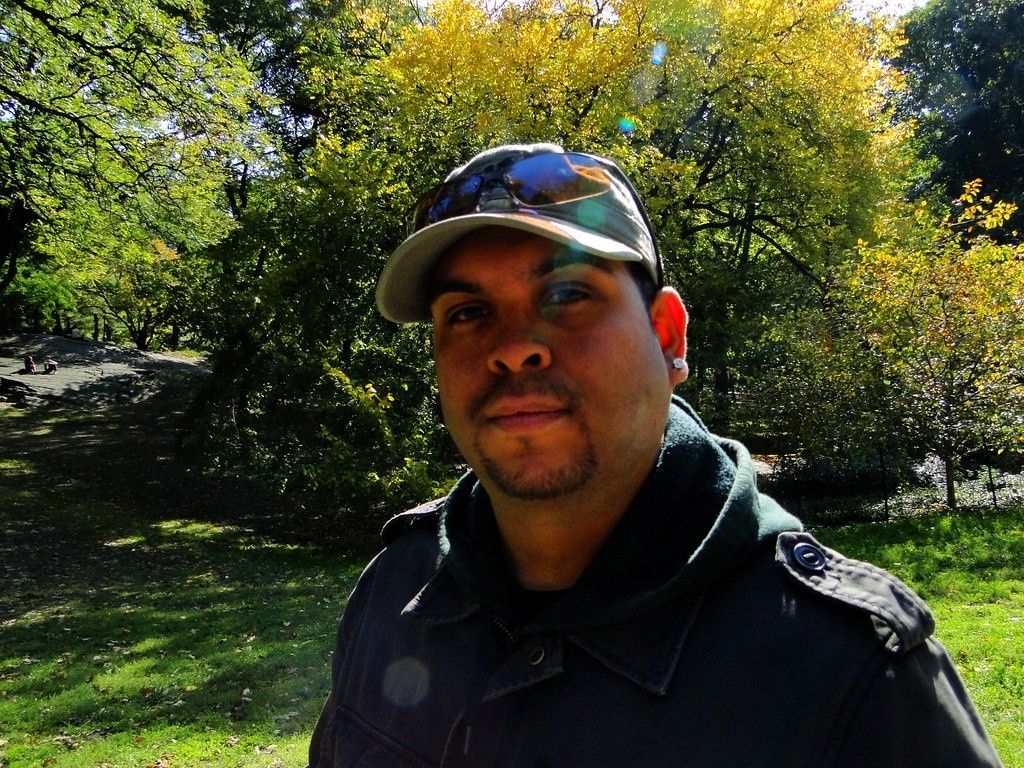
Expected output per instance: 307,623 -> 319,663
306,141 -> 1007,768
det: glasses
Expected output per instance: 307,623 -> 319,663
402,150 -> 670,288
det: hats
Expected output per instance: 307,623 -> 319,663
377,141 -> 665,324
